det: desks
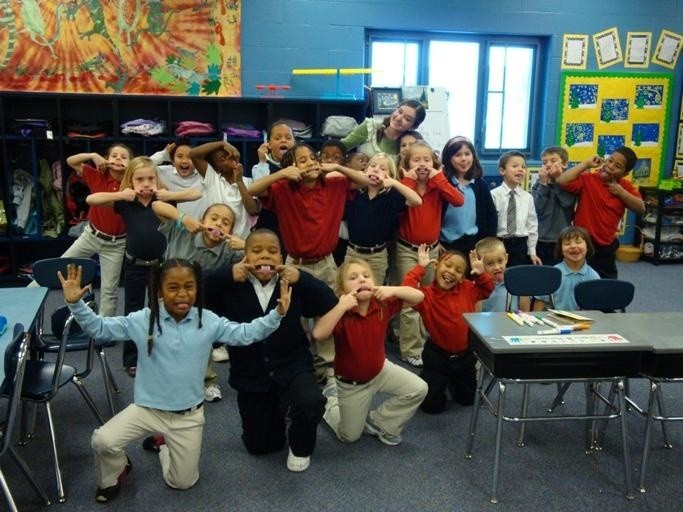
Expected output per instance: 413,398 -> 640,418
600,312 -> 683,495
461,310 -> 654,504
2,286 -> 52,436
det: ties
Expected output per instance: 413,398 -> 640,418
507,191 -> 517,237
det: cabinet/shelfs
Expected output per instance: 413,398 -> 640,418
633,185 -> 683,266
0,91 -> 372,289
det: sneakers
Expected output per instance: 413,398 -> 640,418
364,409 -> 402,447
142,435 -> 163,453
212,346 -> 229,363
286,445 -> 311,473
127,366 -> 137,378
401,355 -> 423,368
203,380 -> 223,403
96,454 -> 133,504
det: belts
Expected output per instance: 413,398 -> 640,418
125,251 -> 159,266
347,242 -> 387,254
168,402 -> 203,415
397,238 -> 439,253
291,254 -> 325,266
335,376 -> 366,386
89,224 -> 126,242
432,346 -> 464,362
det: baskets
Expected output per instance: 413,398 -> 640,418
615,224 -> 644,263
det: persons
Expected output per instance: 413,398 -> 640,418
533,225 -> 601,313
531,147 -> 577,269
398,130 -> 424,159
347,150 -> 371,172
400,242 -> 496,417
312,258 -> 429,446
27,142 -> 169,348
320,138 -> 347,168
86,157 -> 202,377
252,120 -> 296,183
188,140 -> 262,362
490,150 -> 543,269
555,146 -> 645,281
202,228 -> 340,472
340,152 -> 422,287
56,257 -> 293,503
151,200 -> 246,403
247,143 -> 370,388
474,238 -> 530,313
440,135 -> 498,256
340,99 -> 426,162
145,141 -> 203,204
397,140 -> 464,367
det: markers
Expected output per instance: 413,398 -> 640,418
506,309 -> 591,335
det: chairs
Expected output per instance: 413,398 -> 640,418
1,327 -> 51,512
0,292 -> 112,504
26,257 -> 121,440
548,278 -> 674,457
479,265 -> 606,455
